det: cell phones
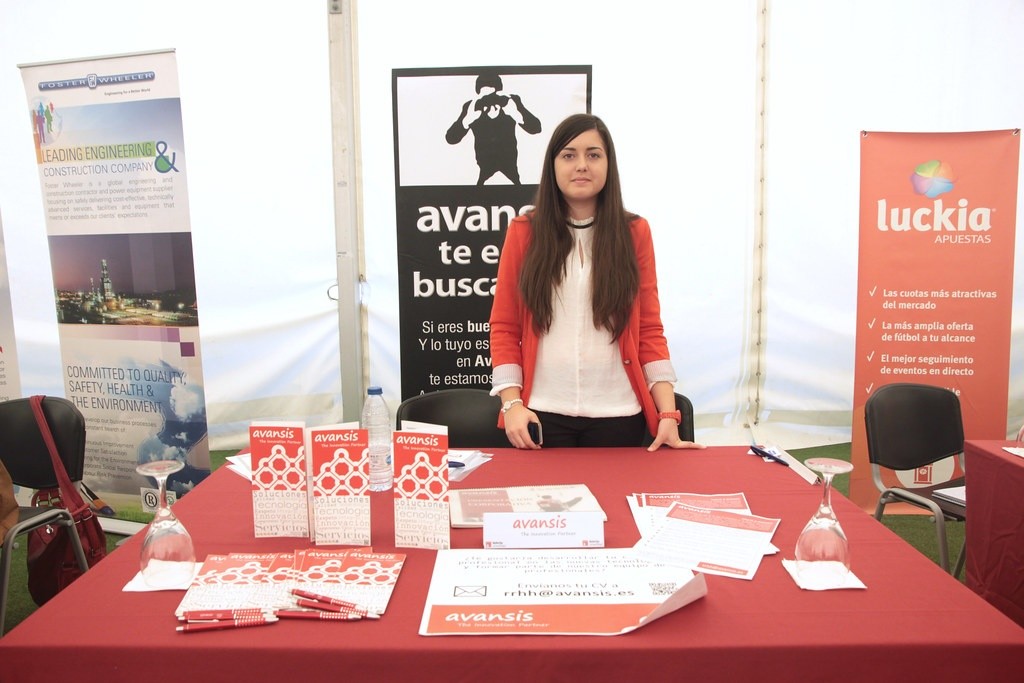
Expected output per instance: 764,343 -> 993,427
528,421 -> 540,445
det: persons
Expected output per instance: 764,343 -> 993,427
489,113 -> 707,452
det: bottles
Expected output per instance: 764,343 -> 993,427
362,387 -> 392,492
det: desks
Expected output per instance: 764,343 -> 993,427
962,439 -> 1024,629
0,443 -> 1023,683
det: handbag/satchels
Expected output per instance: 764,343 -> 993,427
27,395 -> 107,608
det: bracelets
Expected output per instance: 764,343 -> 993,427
658,410 -> 681,423
501,399 -> 523,413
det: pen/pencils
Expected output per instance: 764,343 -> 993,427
447,461 -> 465,467
175,587 -> 381,633
750,444 -> 789,467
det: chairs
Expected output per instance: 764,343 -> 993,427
0,397 -> 89,638
864,382 -> 970,581
396,390 -> 517,451
641,390 -> 694,445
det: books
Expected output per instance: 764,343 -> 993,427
174,547 -> 407,617
449,484 -> 607,528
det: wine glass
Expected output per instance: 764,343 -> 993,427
791,456 -> 853,574
135,461 -> 203,584
